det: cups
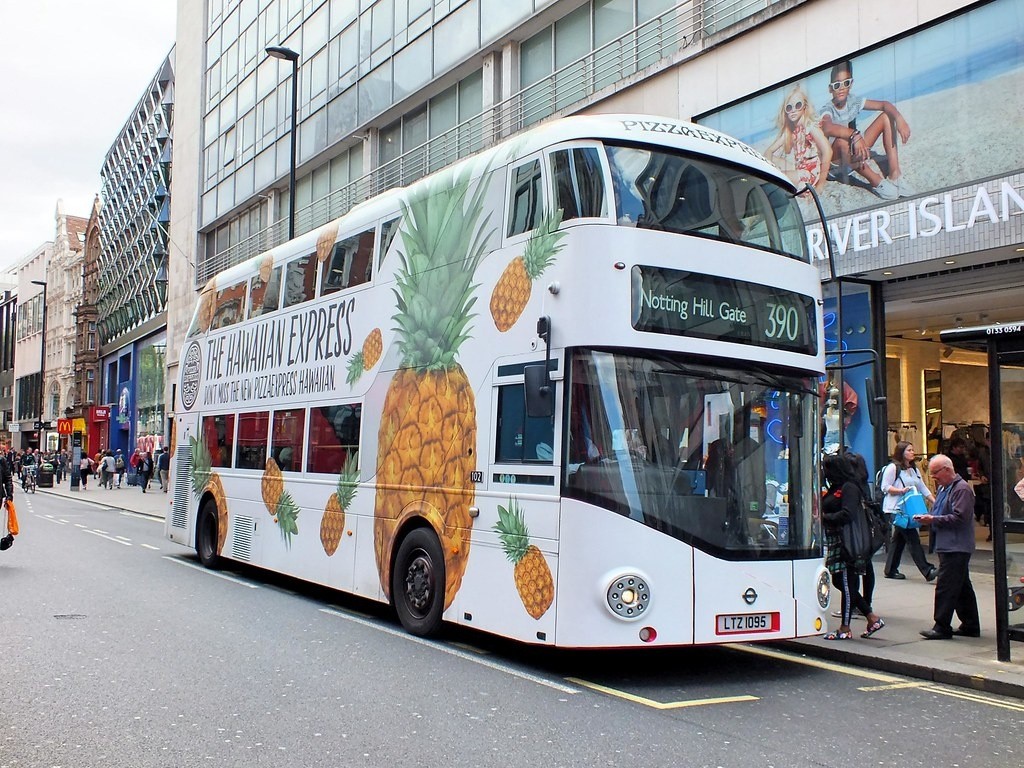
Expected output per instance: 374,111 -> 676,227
967,468 -> 972,474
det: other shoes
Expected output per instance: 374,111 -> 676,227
858,609 -> 865,616
986,535 -> 992,542
926,568 -> 939,580
149,485 -> 150,489
861,618 -> 885,638
831,608 -> 858,618
823,629 -> 852,640
919,629 -> 952,639
143,489 -> 145,493
952,628 -> 980,637
885,572 -> 905,579
160,487 -> 163,489
82,477 -> 120,489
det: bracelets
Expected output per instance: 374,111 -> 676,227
851,136 -> 863,145
848,129 -> 859,141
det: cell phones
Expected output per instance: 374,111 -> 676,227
913,515 -> 921,520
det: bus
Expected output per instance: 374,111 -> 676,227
161,114 -> 888,647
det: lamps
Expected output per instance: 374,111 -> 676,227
956,317 -> 964,328
943,346 -> 952,359
980,313 -> 990,325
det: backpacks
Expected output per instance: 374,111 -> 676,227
875,462 -> 918,508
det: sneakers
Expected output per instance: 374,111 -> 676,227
886,176 -> 918,197
870,179 -> 900,200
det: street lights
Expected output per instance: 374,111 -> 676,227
31,280 -> 48,454
266,46 -> 301,241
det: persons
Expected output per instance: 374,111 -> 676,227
879,443 -> 939,581
535,401 -> 647,466
636,417 -> 680,470
805,364 -> 857,521
819,61 -> 917,200
703,406 -> 767,535
912,453 -> 983,640
819,453 -> 884,641
0,448 -> 171,494
766,78 -> 831,204
0,455 -> 13,550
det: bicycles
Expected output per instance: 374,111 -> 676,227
21,465 -> 36,493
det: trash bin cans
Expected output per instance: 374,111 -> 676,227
38,463 -> 54,488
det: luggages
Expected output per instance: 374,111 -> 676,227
127,467 -> 137,486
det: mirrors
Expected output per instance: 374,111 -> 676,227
924,369 -> 942,461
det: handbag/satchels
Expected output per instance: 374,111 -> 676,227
839,480 -> 887,570
96,460 -> 108,472
116,454 -> 124,468
892,486 -> 928,529
0,497 -> 18,551
87,458 -> 92,472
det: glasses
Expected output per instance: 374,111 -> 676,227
785,101 -> 804,113
830,78 -> 852,90
